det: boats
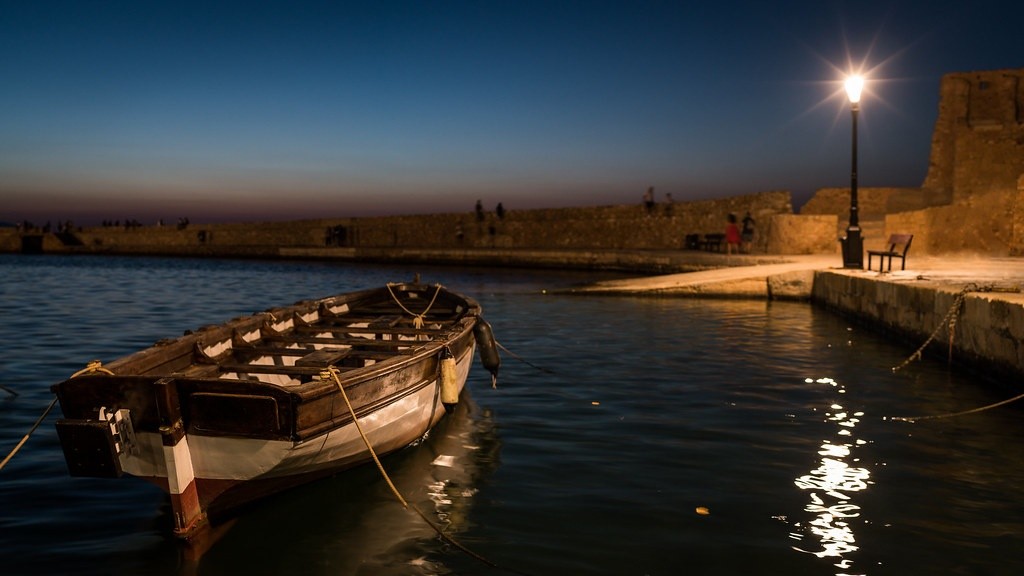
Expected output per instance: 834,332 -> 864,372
50,274 -> 503,514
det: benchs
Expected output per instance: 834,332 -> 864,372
698,242 -> 720,250
869,233 -> 913,273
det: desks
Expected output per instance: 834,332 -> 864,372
704,233 -> 727,251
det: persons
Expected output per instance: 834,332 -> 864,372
475,200 -> 483,221
663,193 -> 677,217
740,212 -> 756,255
156,219 -> 164,225
325,225 -> 347,248
455,223 -> 465,249
487,223 -> 497,248
102,219 -> 137,227
642,186 -> 656,215
178,216 -> 189,224
726,214 -> 740,256
15,218 -> 73,233
197,229 -> 212,243
496,202 -> 507,221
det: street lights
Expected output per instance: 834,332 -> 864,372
838,72 -> 868,267
